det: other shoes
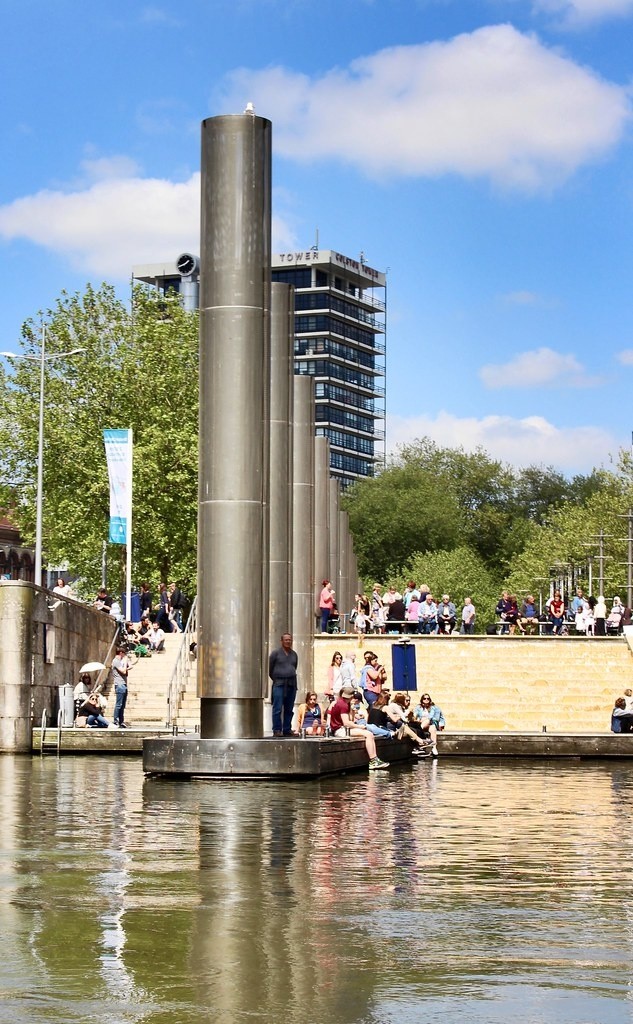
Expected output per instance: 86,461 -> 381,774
119,723 -> 126,728
284,731 -> 299,737
321,626 -> 557,635
319,720 -> 326,736
273,731 -> 284,737
86,724 -> 92,727
395,723 -> 405,740
312,719 -> 319,735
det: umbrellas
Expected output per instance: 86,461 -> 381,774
79,662 -> 106,673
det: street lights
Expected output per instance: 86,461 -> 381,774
0,348 -> 90,588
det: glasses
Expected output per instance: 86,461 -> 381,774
354,691 -> 360,698
423,697 -> 430,700
405,700 -> 410,702
335,657 -> 342,659
365,656 -> 369,659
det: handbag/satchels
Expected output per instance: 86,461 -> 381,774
538,614 -> 547,622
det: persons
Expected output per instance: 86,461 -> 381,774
350,580 -> 455,648
111,647 -> 133,728
124,616 -> 166,658
140,583 -> 182,633
545,589 -> 564,636
53,578 -> 71,598
93,588 -> 113,614
73,673 -> 119,730
606,596 -> 625,628
571,589 -> 606,636
319,579 -> 338,635
460,597 -> 476,635
495,590 -> 539,636
297,651 -> 445,770
269,633 -> 299,738
611,689 -> 633,734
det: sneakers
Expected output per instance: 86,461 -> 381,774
368,761 -> 387,769
377,759 -> 389,767
411,738 -> 438,757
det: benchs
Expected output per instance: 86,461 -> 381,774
494,620 -> 595,634
384,619 -> 457,634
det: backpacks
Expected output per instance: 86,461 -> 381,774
178,593 -> 187,607
166,591 -> 171,602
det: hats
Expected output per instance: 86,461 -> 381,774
374,583 -> 381,589
354,691 -> 362,700
342,687 -> 354,698
